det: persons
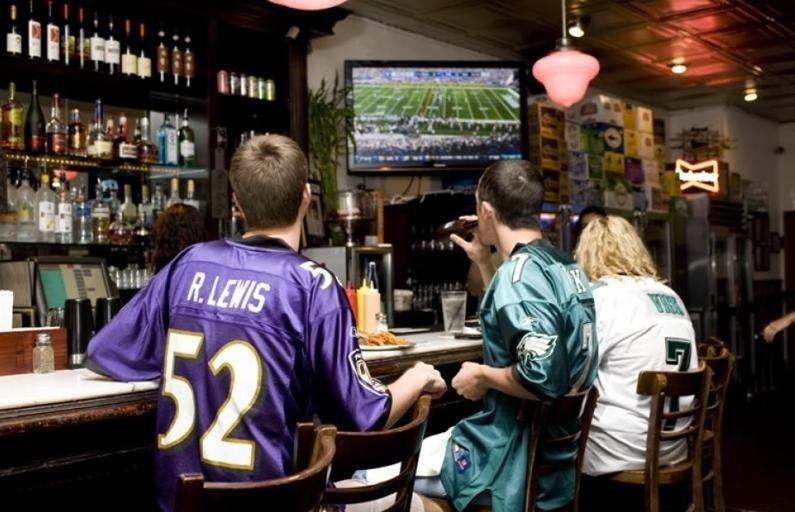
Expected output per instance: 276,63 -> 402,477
85,133 -> 454,512
763,312 -> 795,344
571,214 -> 700,479
577,205 -> 606,232
152,204 -> 205,273
350,158 -> 599,512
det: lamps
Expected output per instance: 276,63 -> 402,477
530,0 -> 602,109
564,12 -> 592,39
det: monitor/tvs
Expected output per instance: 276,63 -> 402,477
345,61 -> 527,175
29,256 -> 112,327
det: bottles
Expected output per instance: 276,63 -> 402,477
367,262 -> 379,291
15,335 -> 25,366
343,280 -> 388,334
409,224 -> 454,251
63,295 -> 124,369
228,129 -> 269,235
411,280 -> 462,310
0,0 -> 196,87
0,77 -> 197,168
436,218 -> 480,243
1,159 -> 198,244
210,147 -> 231,219
45,306 -> 64,326
32,333 -> 55,375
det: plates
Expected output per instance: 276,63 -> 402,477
360,342 -> 417,353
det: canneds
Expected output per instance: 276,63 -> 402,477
216,70 -> 277,102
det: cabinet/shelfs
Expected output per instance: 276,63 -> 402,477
0,53 -> 214,257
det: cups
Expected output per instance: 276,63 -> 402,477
112,263 -> 154,289
393,289 -> 414,312
440,290 -> 466,334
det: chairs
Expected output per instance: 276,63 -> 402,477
698,333 -> 726,361
427,381 -> 602,512
288,392 -> 431,512
168,422 -> 340,511
602,359 -> 711,512
696,347 -> 736,511
